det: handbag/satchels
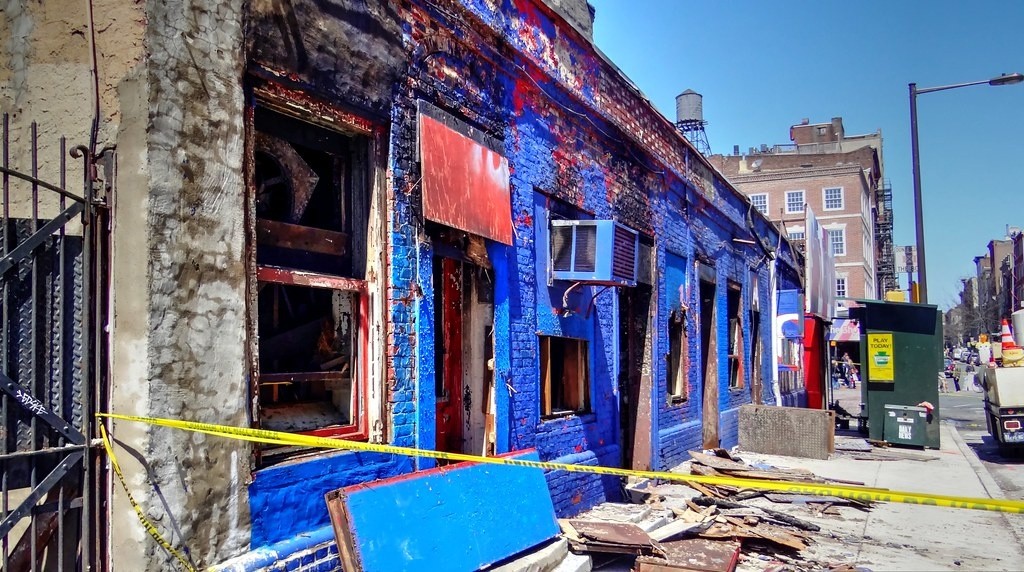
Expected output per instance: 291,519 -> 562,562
849,368 -> 858,375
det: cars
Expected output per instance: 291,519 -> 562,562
951,347 -> 980,365
943,358 -> 956,377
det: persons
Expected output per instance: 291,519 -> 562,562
841,351 -> 858,388
952,360 -> 961,391
965,359 -> 975,391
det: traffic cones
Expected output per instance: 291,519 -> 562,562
1000,318 -> 1017,351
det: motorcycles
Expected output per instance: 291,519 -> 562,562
974,371 -> 1024,458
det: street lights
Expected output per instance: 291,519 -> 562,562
906,70 -> 1024,305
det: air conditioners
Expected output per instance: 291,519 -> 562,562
550,218 -> 640,287
